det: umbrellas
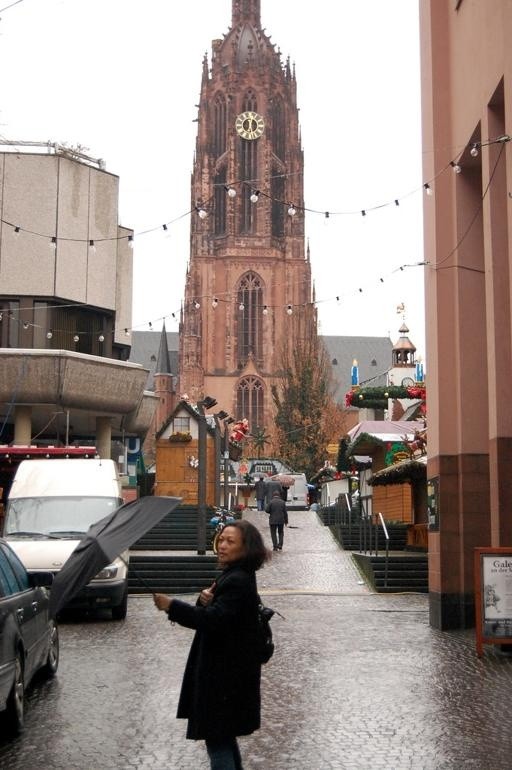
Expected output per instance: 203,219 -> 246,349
272,474 -> 296,488
251,472 -> 267,478
49,494 -> 183,616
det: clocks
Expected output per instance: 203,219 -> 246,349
234,111 -> 265,142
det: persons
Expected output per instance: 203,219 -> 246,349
264,492 -> 288,552
254,477 -> 289,512
151,521 -> 272,769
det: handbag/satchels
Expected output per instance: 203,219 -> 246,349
258,603 -> 275,663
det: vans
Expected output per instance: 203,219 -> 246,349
1,456 -> 130,621
262,471 -> 310,511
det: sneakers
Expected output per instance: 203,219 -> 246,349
273,547 -> 278,551
277,545 -> 282,549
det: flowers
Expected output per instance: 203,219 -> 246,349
169,432 -> 190,439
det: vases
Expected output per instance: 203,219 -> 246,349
170,436 -> 191,441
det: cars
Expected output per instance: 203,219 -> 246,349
0,539 -> 60,731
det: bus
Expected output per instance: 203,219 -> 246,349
0,442 -> 100,508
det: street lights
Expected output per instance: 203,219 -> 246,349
197,396 -> 235,556
